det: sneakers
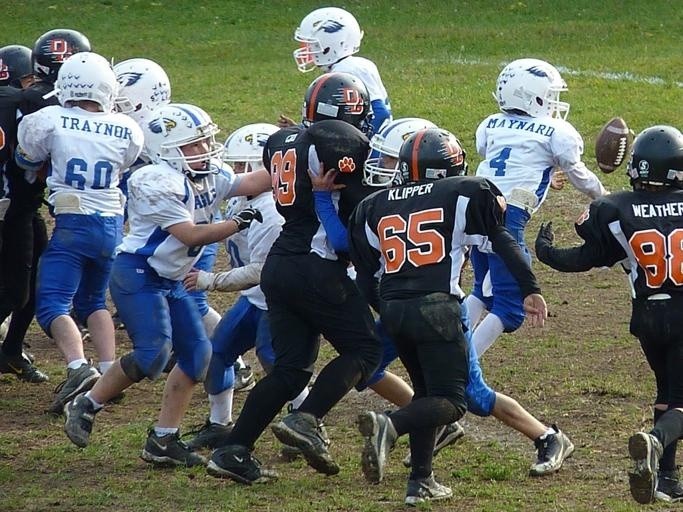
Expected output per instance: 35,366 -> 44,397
629,432 -> 663,504
656,476 -> 683,503
530,424 -> 574,476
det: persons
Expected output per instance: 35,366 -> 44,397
535,125 -> 682,506
459,58 -> 611,363
0,6 -> 574,504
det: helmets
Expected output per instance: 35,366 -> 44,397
490,58 -> 570,120
627,125 -> 683,193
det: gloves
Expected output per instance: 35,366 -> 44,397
232,208 -> 262,230
535,222 -> 554,244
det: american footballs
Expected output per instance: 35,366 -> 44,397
594,116 -> 631,173
